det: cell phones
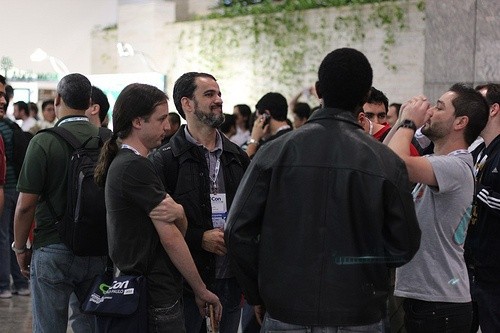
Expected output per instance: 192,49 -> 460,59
262,113 -> 270,129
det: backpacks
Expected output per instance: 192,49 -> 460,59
34,126 -> 116,249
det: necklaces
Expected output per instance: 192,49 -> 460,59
367,118 -> 373,134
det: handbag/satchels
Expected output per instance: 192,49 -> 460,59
79,273 -> 143,318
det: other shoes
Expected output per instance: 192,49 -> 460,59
0,288 -> 12,298
10,282 -> 30,295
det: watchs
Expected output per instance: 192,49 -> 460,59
12,242 -> 26,253
397,119 -> 416,134
247,138 -> 259,145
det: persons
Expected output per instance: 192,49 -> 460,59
94,83 -> 222,333
464,82 -> 500,333
86,85 -> 110,128
360,86 -> 434,191
11,74 -> 110,333
382,83 -> 490,333
216,87 -> 321,161
0,74 -> 57,297
150,71 -> 249,333
225,46 -> 421,333
